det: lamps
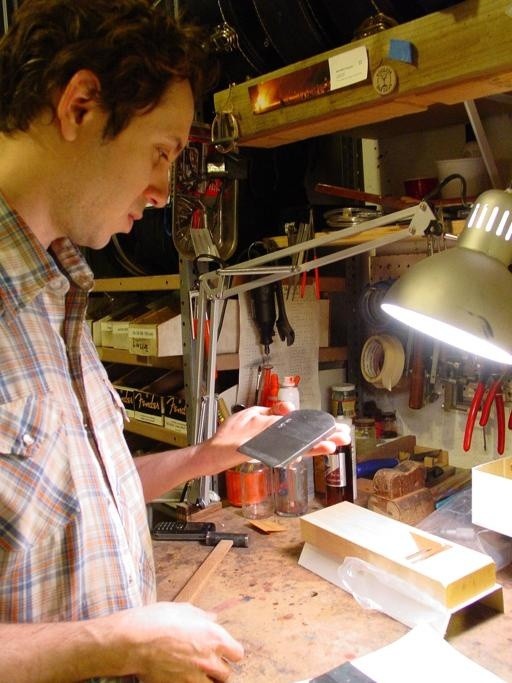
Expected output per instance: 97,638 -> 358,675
183,172 -> 511,511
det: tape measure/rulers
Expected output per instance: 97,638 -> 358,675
173,539 -> 233,603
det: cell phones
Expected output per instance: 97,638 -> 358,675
151,521 -> 215,542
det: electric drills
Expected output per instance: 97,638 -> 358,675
249,263 -> 276,366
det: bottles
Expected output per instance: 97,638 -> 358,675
240,462 -> 275,519
256,364 -> 300,415
314,382 -> 397,507
275,457 -> 307,518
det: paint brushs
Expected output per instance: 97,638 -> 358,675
255,366 -> 262,406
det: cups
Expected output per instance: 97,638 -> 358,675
435,155 -> 488,199
225,470 -> 271,506
403,174 -> 439,199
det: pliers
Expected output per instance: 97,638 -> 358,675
479,366 -> 512,430
463,357 -> 505,455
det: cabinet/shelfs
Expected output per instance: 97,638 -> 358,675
84,270 -> 192,520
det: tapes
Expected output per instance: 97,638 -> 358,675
359,279 -> 399,329
360,334 -> 405,392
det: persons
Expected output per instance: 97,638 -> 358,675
0,2 -> 352,682
